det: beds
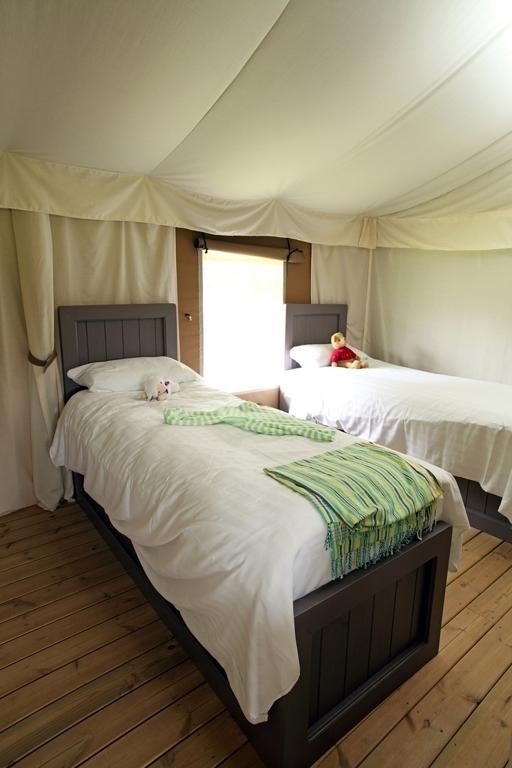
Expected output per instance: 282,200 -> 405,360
47,303 -> 472,768
281,303 -> 512,550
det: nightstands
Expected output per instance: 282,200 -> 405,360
231,384 -> 280,410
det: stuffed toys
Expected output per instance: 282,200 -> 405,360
330,332 -> 369,369
144,376 -> 180,401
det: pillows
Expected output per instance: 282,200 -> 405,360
64,355 -> 202,394
289,341 -> 368,368
